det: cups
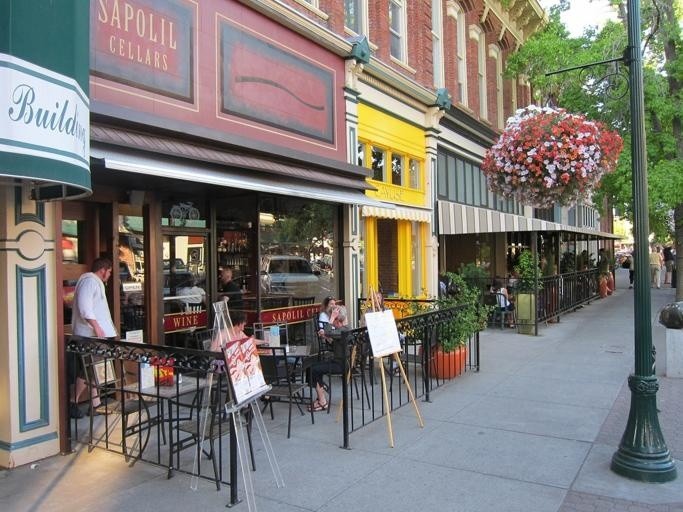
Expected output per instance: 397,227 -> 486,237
289,346 -> 296,353
263,331 -> 269,346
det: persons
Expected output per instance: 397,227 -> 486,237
597,248 -> 611,279
68,258 -> 117,418
628,252 -> 637,288
218,268 -> 244,327
319,296 -> 348,328
177,273 -> 208,334
649,246 -> 664,290
663,242 -> 677,284
577,250 -> 587,270
305,306 -> 352,412
495,282 -> 517,328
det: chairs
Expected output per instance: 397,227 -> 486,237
183,294 -> 413,440
75,351 -> 167,462
169,368 -> 260,491
488,290 -> 514,331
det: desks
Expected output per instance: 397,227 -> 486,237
122,374 -> 207,470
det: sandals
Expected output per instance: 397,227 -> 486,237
308,398 -> 328,413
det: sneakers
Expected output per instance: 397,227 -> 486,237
70,404 -> 83,418
88,405 -> 112,415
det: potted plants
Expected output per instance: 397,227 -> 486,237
403,269 -> 495,380
510,248 -> 545,337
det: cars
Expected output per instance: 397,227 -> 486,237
60,245 -> 324,334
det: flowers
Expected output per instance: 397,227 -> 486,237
480,97 -> 625,213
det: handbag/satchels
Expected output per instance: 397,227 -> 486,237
622,259 -> 632,268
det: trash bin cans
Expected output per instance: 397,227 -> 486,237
663,327 -> 683,377
516,293 -> 534,332
671,264 -> 678,288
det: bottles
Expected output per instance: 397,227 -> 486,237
285,344 -> 289,353
218,231 -> 247,291
178,373 -> 182,384
